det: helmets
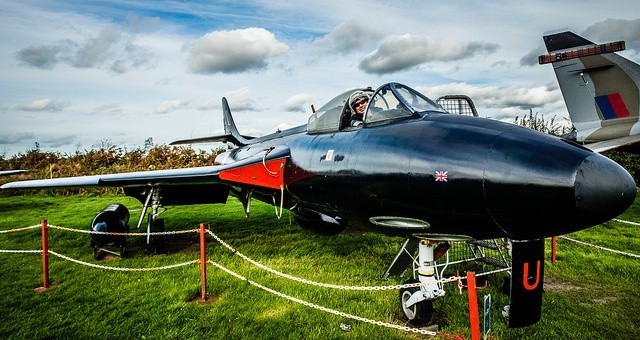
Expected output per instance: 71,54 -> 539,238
348,90 -> 371,111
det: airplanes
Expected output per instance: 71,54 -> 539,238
1,82 -> 636,326
539,31 -> 640,153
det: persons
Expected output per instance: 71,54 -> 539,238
349,92 -> 381,127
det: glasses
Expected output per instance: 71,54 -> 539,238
354,99 -> 369,107
